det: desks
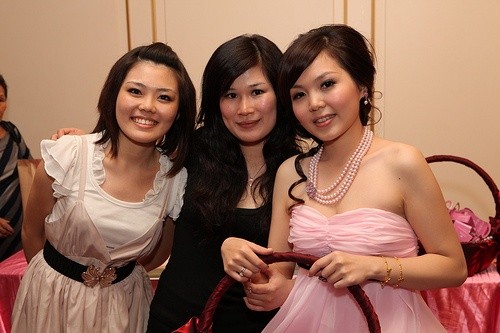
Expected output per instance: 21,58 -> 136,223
0,246 -> 500,333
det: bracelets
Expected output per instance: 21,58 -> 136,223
392,256 -> 403,288
380,256 -> 392,287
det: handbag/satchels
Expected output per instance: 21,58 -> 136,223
17,159 -> 41,214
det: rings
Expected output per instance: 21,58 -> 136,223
239,268 -> 246,277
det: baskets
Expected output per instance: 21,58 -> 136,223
418,154 -> 500,277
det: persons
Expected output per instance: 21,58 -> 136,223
0,74 -> 34,262
49,35 -> 303,333
221,24 -> 468,333
10,42 -> 196,333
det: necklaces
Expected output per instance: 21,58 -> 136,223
248,161 -> 265,187
306,126 -> 373,204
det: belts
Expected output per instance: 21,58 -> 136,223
43,239 -> 136,289
